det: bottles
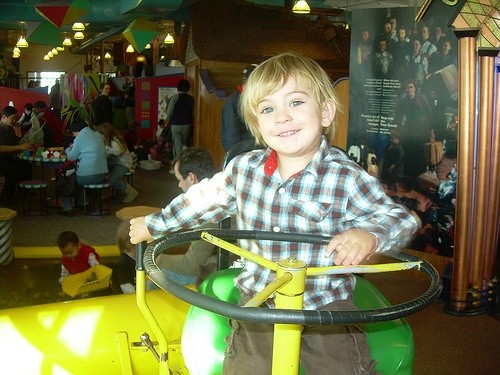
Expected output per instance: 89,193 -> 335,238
36,148 -> 43,158
241,69 -> 247,82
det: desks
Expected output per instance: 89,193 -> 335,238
17,149 -> 114,213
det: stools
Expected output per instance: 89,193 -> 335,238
81,183 -> 110,217
112,169 -> 135,199
20,180 -> 50,216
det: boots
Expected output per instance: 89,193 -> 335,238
122,183 -> 139,203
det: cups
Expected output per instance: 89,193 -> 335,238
42,151 -> 66,159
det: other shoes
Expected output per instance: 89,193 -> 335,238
57,208 -> 74,217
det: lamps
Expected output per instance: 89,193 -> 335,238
164,20 -> 175,43
13,47 -> 21,58
292,0 -> 310,13
43,34 -> 72,61
74,32 -> 84,40
16,21 -> 29,47
72,22 -> 86,30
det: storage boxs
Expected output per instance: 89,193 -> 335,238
138,160 -> 161,170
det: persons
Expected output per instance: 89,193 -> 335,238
348,137 -> 370,166
59,118 -> 108,217
97,122 -> 138,203
111,220 -> 137,296
0,106 -> 36,197
381,129 -> 405,189
27,80 -> 40,88
57,231 -> 101,298
146,147 -> 219,292
17,101 -> 64,145
430,89 -> 443,116
396,78 -> 435,176
128,52 -> 418,375
166,79 -> 194,159
355,16 -> 454,88
220,66 -> 255,154
92,83 -> 112,131
